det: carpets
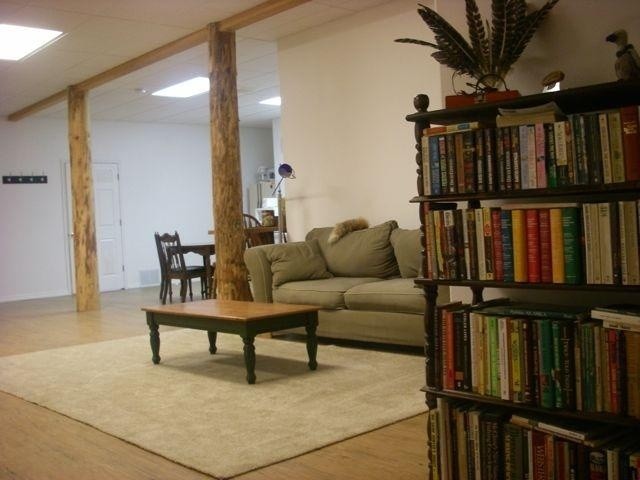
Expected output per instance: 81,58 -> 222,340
0,328 -> 427,480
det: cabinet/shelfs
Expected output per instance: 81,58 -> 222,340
408,76 -> 640,478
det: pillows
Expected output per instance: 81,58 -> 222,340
263,235 -> 332,288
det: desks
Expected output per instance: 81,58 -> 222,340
207,227 -> 278,247
163,244 -> 216,304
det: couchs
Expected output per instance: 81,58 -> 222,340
243,220 -> 425,351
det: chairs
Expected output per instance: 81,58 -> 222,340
155,230 -> 214,300
243,214 -> 262,227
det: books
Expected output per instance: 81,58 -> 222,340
431,298 -> 640,420
420,100 -> 640,197
423,202 -> 640,286
429,397 -> 640,480
